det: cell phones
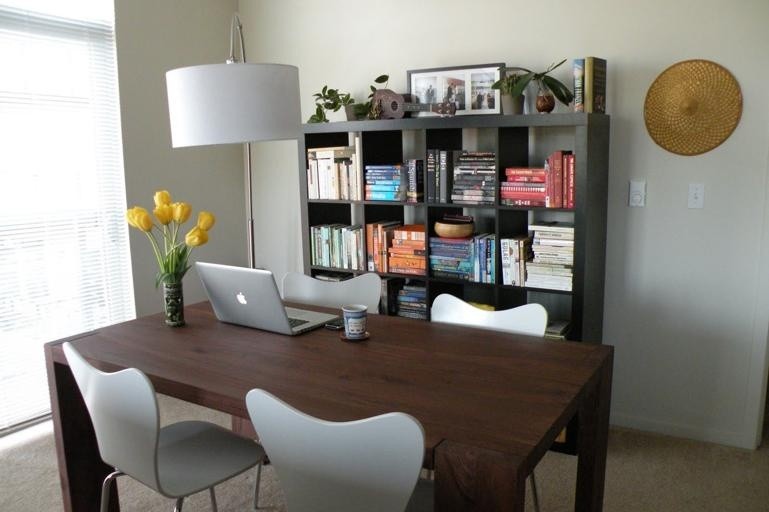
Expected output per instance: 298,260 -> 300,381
325,318 -> 345,330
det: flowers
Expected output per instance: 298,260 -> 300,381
125,189 -> 216,290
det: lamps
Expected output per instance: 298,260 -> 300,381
164,14 -> 303,267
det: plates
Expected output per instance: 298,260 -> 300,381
339,331 -> 370,341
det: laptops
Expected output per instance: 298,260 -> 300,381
195,262 -> 339,336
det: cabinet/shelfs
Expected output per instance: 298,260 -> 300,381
296,113 -> 611,458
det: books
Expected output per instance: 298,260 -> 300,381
427,149 -> 495,205
311,224 -> 363,271
316,271 -> 353,282
396,280 -> 427,320
429,232 -> 495,284
306,146 -> 362,201
500,221 -> 575,291
500,151 -> 574,209
366,220 -> 427,276
543,320 -> 573,340
363,159 -> 424,203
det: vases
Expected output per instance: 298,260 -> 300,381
161,280 -> 187,327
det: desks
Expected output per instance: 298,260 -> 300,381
42,299 -> 614,511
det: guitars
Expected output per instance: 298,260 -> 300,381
371,90 -> 456,119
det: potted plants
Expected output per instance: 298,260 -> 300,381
307,74 -> 389,124
491,59 -> 573,114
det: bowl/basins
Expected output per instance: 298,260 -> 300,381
433,219 -> 476,239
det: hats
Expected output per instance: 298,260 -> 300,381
642,60 -> 742,157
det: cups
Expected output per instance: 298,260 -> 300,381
341,304 -> 368,339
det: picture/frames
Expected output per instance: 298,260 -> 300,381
406,62 -> 506,117
